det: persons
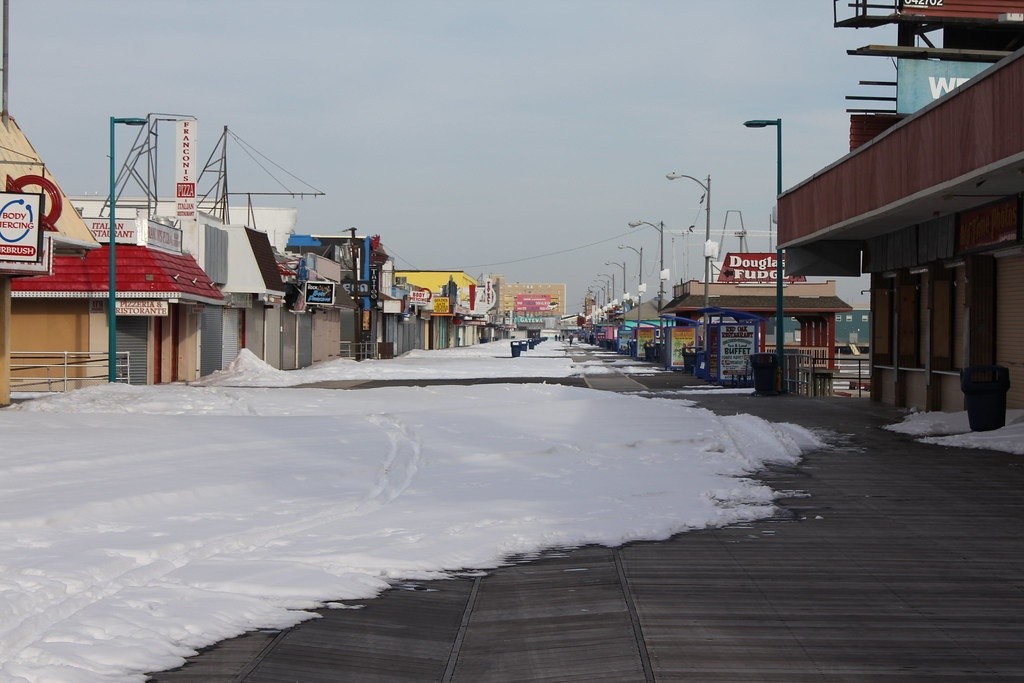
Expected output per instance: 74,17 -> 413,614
569,333 -> 574,345
589,333 -> 594,345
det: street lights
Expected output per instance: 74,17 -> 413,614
109,116 -> 150,385
586,289 -> 598,325
626,220 -> 664,365
604,260 -> 625,330
744,118 -> 785,395
591,285 -> 605,332
593,279 -> 610,350
666,172 -> 711,351
597,273 -> 615,350
618,245 -> 642,328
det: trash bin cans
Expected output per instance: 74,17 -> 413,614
643,342 -> 656,362
682,347 -> 704,374
605,339 -> 612,350
749,353 -> 779,397
960,365 -> 1011,432
